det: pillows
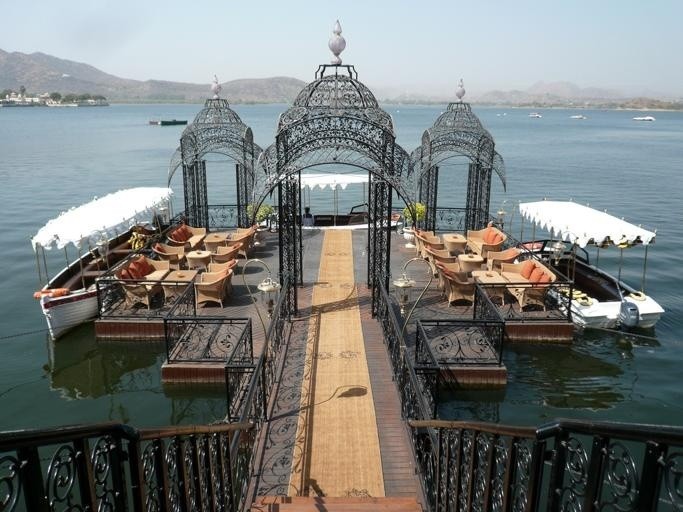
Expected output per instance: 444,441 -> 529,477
121,256 -> 151,285
169,225 -> 193,242
521,260 -> 551,292
155,243 -> 165,254
482,227 -> 503,244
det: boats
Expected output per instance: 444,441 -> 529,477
262,212 -> 404,232
30,188 -> 170,341
42,326 -> 171,402
515,199 -> 664,332
148,119 -> 188,125
527,112 -> 653,121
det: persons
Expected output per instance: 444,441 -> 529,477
303,206 -> 315,226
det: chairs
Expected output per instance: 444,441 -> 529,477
485,247 -> 520,274
193,225 -> 259,309
150,242 -> 185,271
413,230 -> 477,308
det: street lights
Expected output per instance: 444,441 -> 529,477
391,257 -> 432,413
242,253 -> 281,423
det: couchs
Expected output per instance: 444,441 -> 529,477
166,223 -> 207,250
499,258 -> 557,312
113,255 -> 170,310
466,226 -> 507,258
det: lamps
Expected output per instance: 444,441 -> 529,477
242,258 -> 282,362
393,257 -> 434,344
